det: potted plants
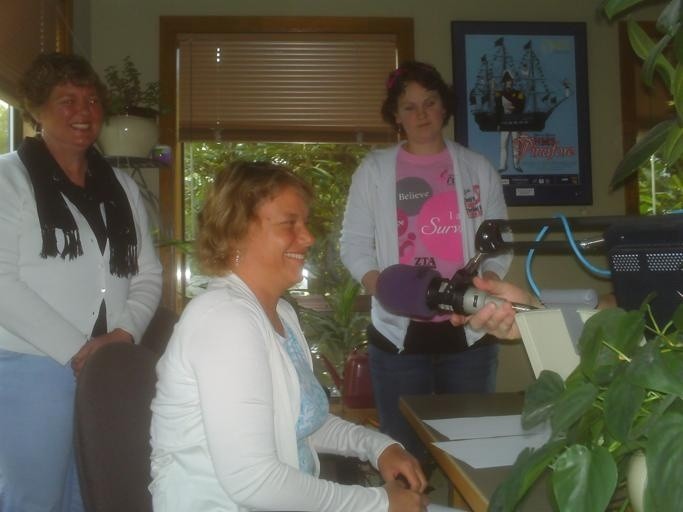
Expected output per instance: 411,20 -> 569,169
91,55 -> 173,158
483,290 -> 683,511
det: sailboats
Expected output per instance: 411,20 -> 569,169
469,37 -> 570,132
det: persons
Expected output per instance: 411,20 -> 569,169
336,61 -> 515,472
144,157 -> 454,511
0,49 -> 169,511
443,274 -> 618,343
494,70 -> 525,173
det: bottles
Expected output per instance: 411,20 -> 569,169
152,145 -> 172,163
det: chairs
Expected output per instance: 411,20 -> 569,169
76,338 -> 158,510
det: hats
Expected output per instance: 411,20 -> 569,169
383,60 -> 441,89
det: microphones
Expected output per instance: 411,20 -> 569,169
375,264 -> 539,320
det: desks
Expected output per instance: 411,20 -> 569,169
398,390 -> 632,512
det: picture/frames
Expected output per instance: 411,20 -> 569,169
450,15 -> 596,207
